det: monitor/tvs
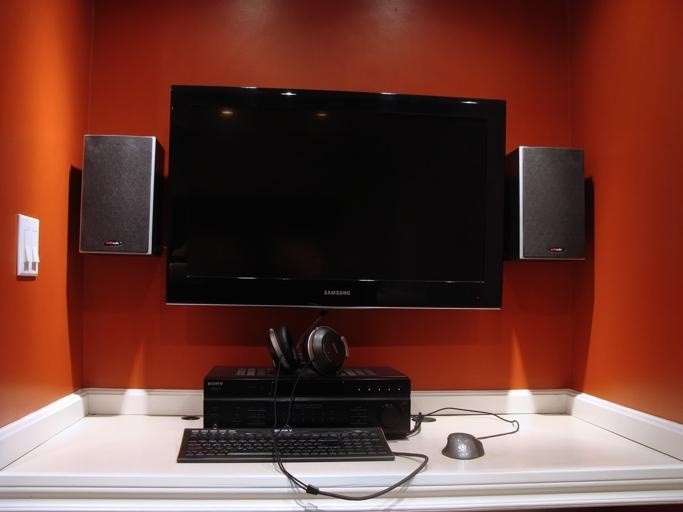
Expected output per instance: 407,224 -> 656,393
165,84 -> 508,311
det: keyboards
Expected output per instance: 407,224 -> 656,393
176,428 -> 395,464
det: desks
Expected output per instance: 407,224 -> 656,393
0,387 -> 682,512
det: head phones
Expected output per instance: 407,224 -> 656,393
264,322 -> 350,376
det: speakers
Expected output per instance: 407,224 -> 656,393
507,144 -> 586,263
79,133 -> 165,258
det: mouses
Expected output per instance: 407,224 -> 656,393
441,432 -> 485,460
